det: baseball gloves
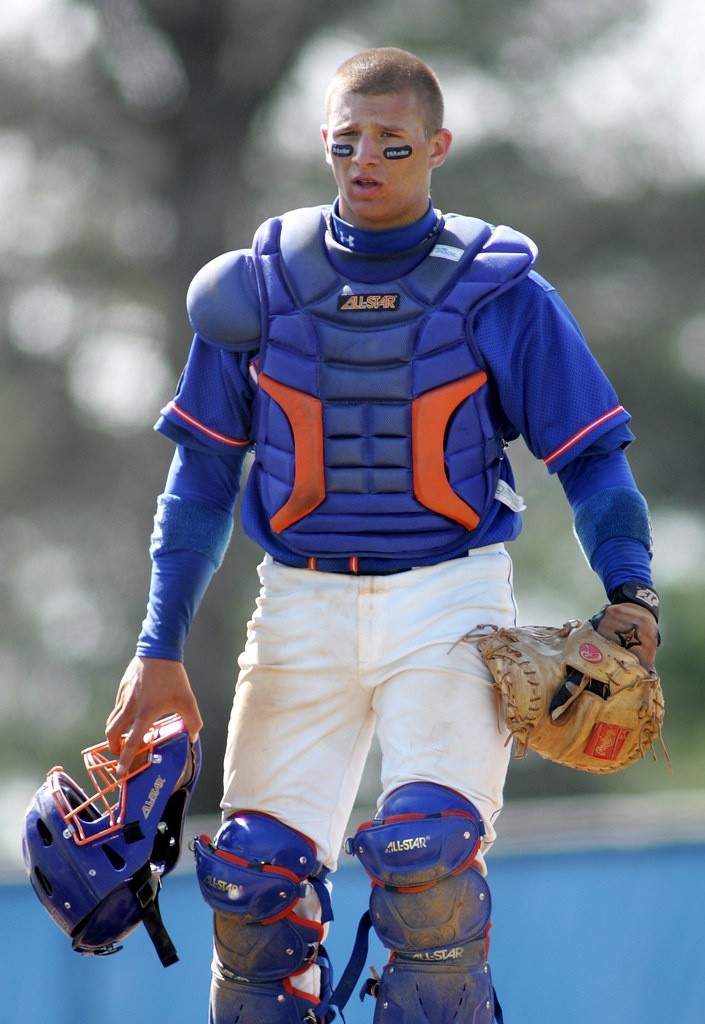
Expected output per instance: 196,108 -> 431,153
443,580 -> 674,776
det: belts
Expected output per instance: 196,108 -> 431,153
273,549 -> 469,576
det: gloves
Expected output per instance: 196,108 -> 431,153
549,582 -> 661,721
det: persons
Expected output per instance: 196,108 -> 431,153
101,49 -> 658,1024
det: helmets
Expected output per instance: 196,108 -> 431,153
22,714 -> 203,956
